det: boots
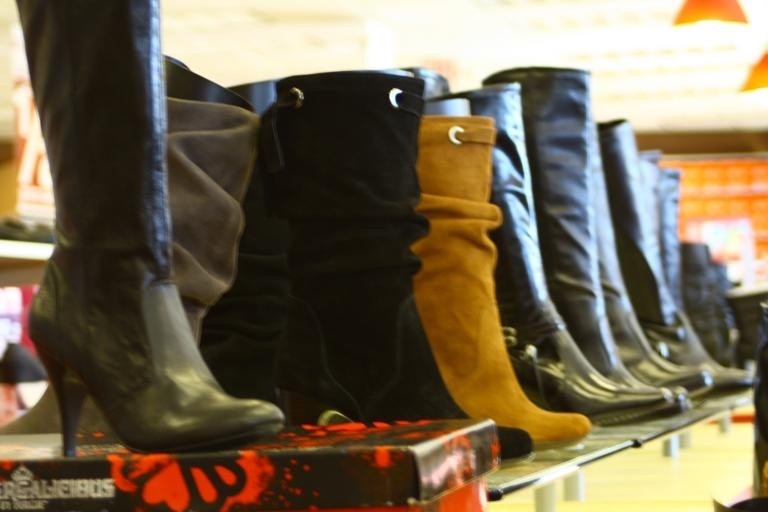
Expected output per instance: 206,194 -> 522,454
587,119 -> 713,399
276,70 -> 534,462
16,0 -> 285,457
426,81 -> 673,425
482,67 -> 689,422
166,96 -> 259,348
597,119 -> 756,401
727,292 -> 768,369
411,115 -> 592,452
